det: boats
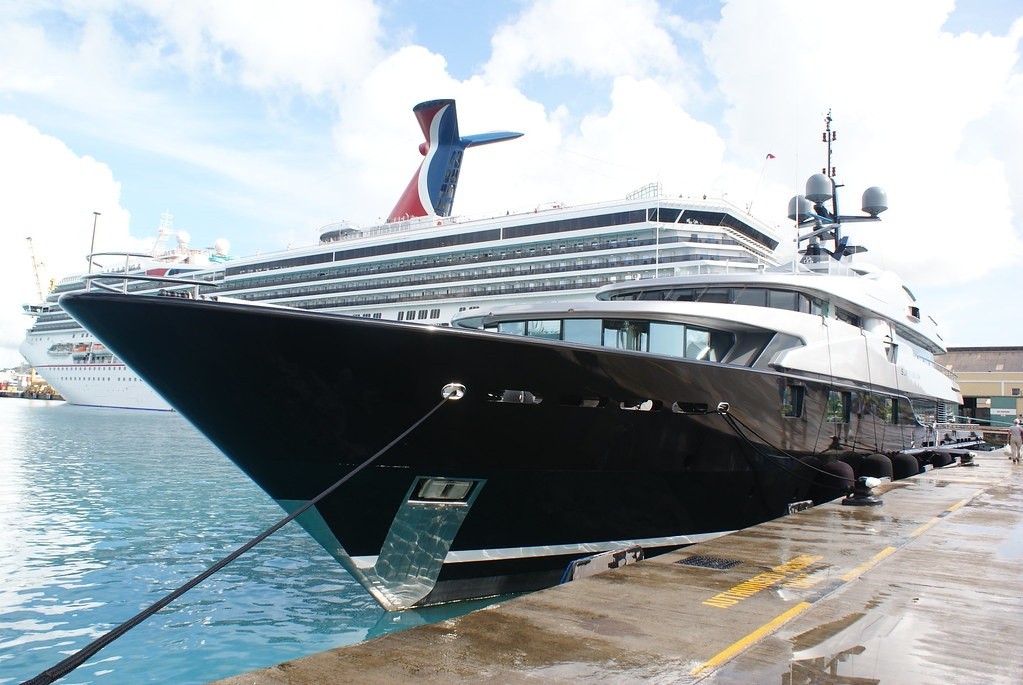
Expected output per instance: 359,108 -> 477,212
16,100 -> 783,411
56,105 -> 988,612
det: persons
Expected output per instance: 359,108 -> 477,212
940,433 -> 955,445
1007,419 -> 1023,462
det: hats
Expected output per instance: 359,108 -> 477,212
1014,419 -> 1018,422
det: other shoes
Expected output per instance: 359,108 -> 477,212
1013,460 -> 1016,462
1017,459 -> 1019,462
